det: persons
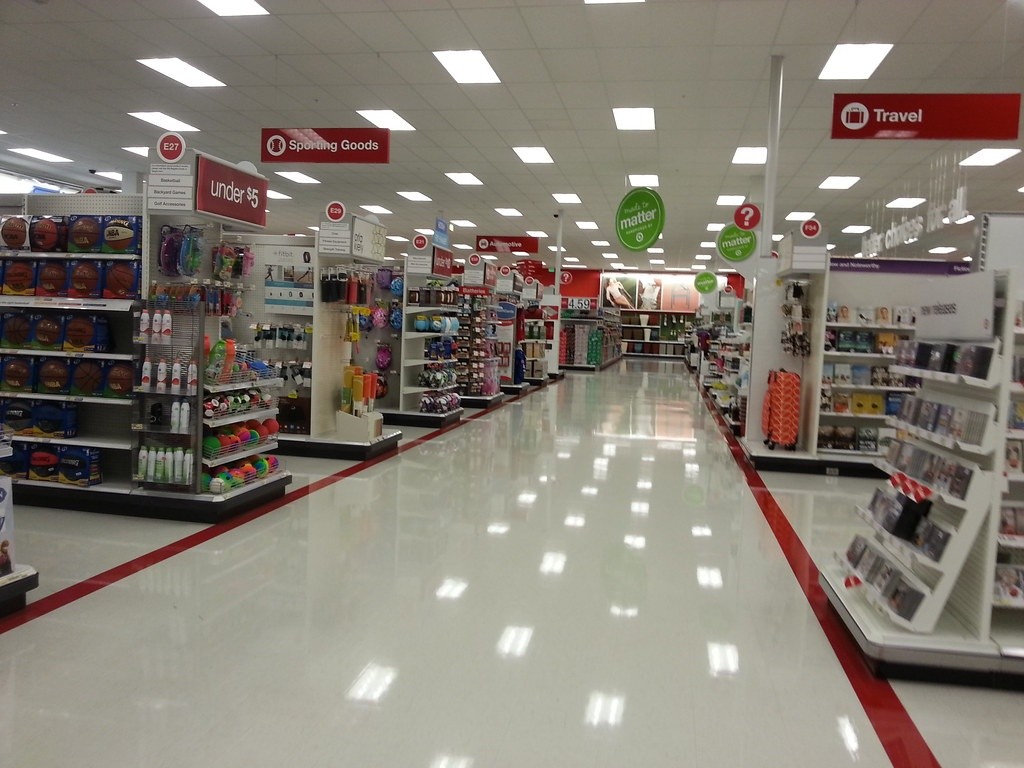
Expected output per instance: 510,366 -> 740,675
637,279 -> 661,310
606,277 -> 634,309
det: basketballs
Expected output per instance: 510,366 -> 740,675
1,217 -> 142,489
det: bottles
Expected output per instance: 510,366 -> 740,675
156,359 -> 167,393
170,398 -> 179,434
171,359 -> 181,394
137,446 -> 194,485
152,310 -> 161,343
139,309 -> 149,342
161,310 -> 173,343
204,334 -> 236,383
187,360 -> 198,393
141,357 -> 151,390
179,398 -> 190,434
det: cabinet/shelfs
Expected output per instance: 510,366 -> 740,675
376,261 -> 466,428
455,274 -> 505,409
619,303 -> 751,435
517,280 -> 553,384
223,230 -> 403,461
496,274 -> 531,396
551,305 -> 625,371
818,209 -> 1024,682
0,181 -> 293,527
738,230 -> 972,479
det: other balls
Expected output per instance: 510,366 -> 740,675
203,383 -> 281,491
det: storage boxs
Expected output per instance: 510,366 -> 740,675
456,295 -> 500,396
524,308 -> 549,380
817,309 -> 993,619
0,214 -> 144,486
264,280 -> 314,318
0,476 -> 16,575
334,409 -> 384,442
407,286 -> 459,307
560,323 -> 624,367
